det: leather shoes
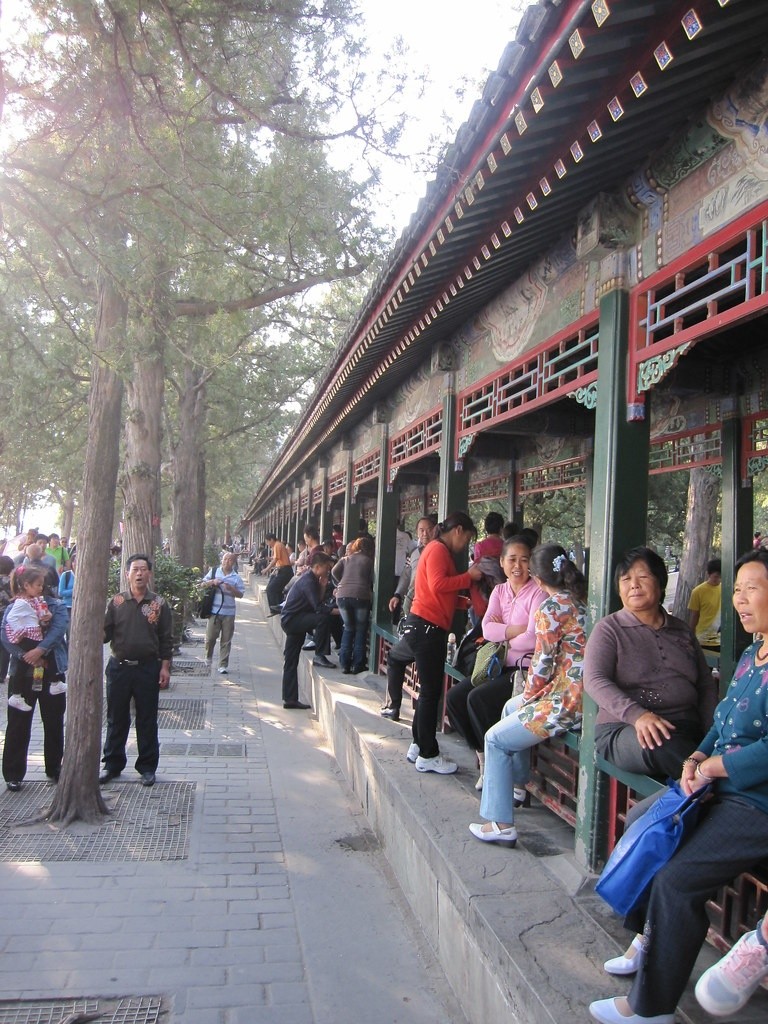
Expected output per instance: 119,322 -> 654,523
302,644 -> 369,675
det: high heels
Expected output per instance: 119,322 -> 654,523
512,787 -> 526,807
468,821 -> 517,848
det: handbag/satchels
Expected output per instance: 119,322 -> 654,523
510,653 -> 534,697
594,777 -> 714,918
471,640 -> 508,687
200,567 -> 217,619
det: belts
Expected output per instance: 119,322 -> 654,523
110,651 -> 158,666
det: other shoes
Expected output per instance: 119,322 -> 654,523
604,937 -> 642,975
283,701 -> 310,709
99,771 -> 120,783
589,996 -> 676,1024
142,773 -> 155,785
218,666 -> 228,674
205,656 -> 212,666
52,775 -> 59,783
381,708 -> 399,721
267,613 -> 276,618
270,605 -> 283,613
475,775 -> 484,790
7,781 -> 21,791
0,677 -> 4,683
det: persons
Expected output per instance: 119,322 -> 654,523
202,510 -> 763,847
98,554 -> 174,786
694,909 -> 768,1015
589,544 -> 768,1024
0,529 -> 121,791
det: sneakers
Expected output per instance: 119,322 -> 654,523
694,930 -> 768,1016
8,696 -> 32,711
49,681 -> 67,696
415,751 -> 457,775
406,743 -> 451,763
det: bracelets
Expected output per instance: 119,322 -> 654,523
682,757 -> 699,769
697,762 -> 715,781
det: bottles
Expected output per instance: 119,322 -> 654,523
31,667 -> 45,692
36,596 -> 49,625
